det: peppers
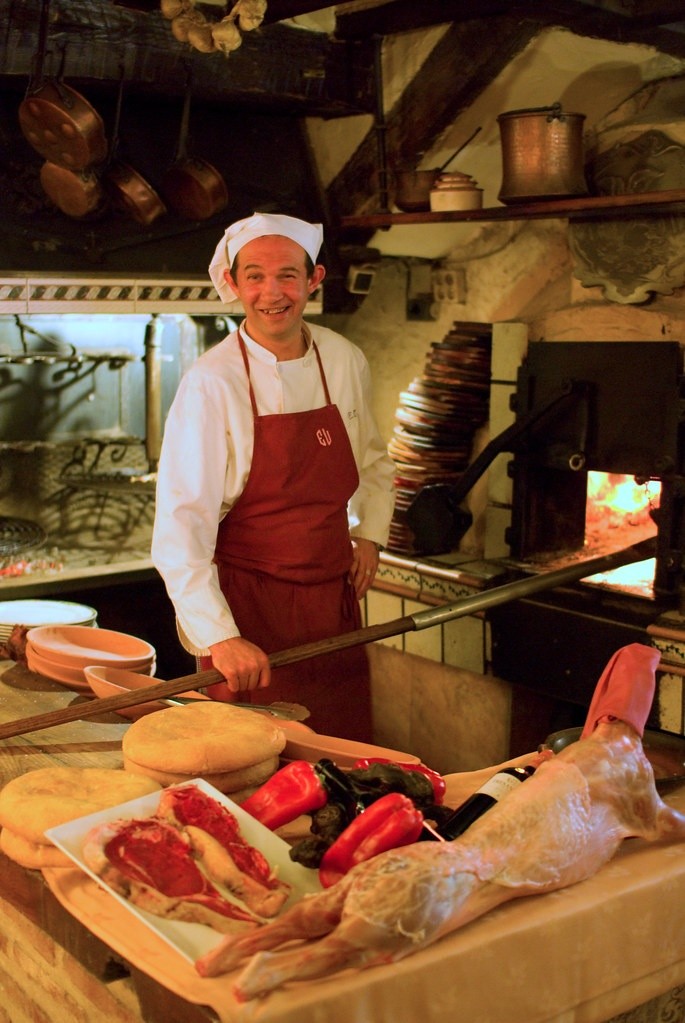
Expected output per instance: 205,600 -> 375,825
240,754 -> 447,888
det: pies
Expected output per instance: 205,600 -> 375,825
0,767 -> 165,870
123,702 -> 287,791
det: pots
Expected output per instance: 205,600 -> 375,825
40,153 -> 103,217
20,32 -> 108,170
167,92 -> 226,220
107,43 -> 167,225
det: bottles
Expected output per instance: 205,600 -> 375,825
314,758 -> 446,844
435,748 -> 554,842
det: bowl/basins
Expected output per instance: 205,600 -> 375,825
394,167 -> 447,212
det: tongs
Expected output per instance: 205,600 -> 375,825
159,698 -> 310,721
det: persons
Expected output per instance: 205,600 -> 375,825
148,212 -> 396,743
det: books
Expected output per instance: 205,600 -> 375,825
382,321 -> 494,555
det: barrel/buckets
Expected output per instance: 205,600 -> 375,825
496,103 -> 586,205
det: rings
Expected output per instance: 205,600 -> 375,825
366,569 -> 371,575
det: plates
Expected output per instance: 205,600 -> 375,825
0,600 -> 213,719
0,514 -> 49,555
537,727 -> 685,785
46,778 -> 325,965
